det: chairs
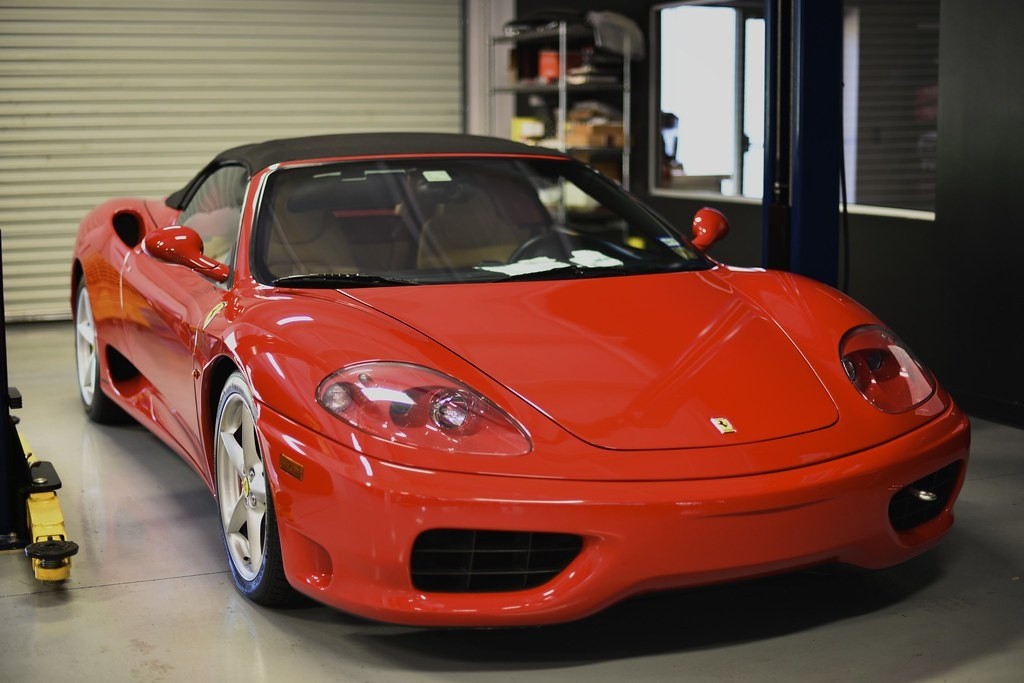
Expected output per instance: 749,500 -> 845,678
418,174 -> 522,270
267,177 -> 360,278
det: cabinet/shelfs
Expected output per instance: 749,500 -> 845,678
487,21 -> 632,192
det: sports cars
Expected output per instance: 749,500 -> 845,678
70,132 -> 971,627
